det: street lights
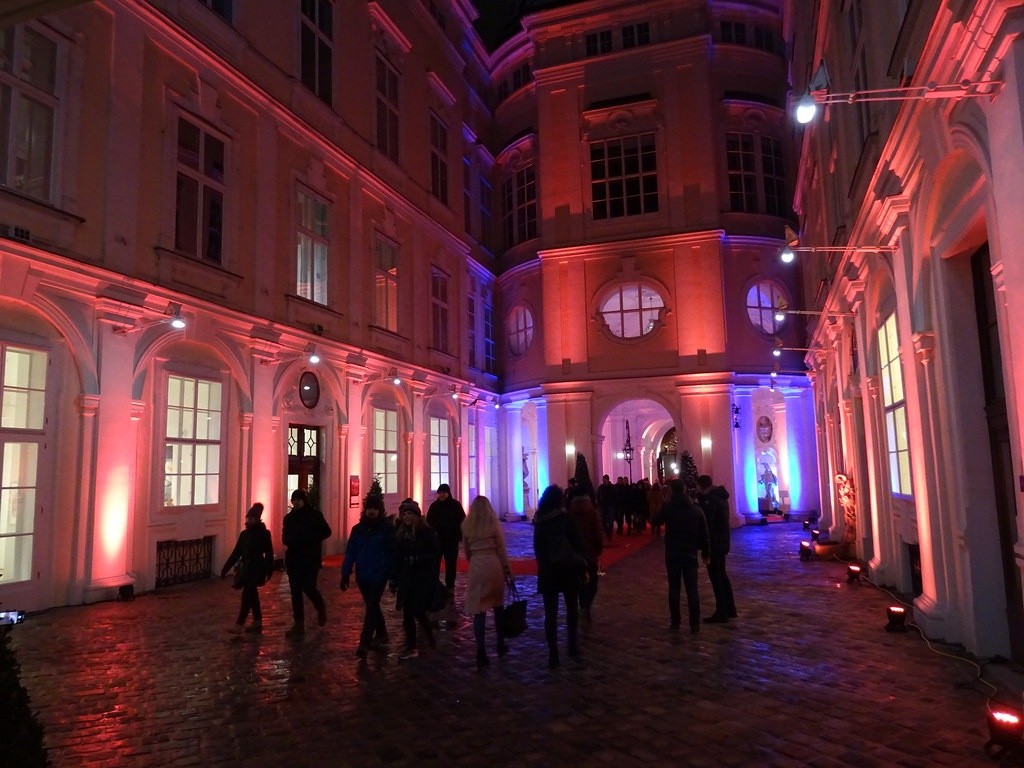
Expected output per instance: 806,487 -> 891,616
622,419 -> 635,486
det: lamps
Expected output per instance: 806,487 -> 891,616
848,565 -> 861,581
986,706 -> 1022,758
804,520 -> 811,529
779,227 -> 899,263
117,585 -> 135,601
473,399 -> 500,410
888,606 -> 907,630
273,558 -> 284,571
789,80 -> 1005,124
800,540 -> 811,559
415,390 -> 458,401
774,295 -> 856,322
116,314 -> 187,330
772,334 -> 828,355
260,352 -> 320,367
623,439 -> 633,464
769,370 -> 811,389
356,374 -> 400,386
812,529 -> 820,539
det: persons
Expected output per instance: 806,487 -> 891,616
760,464 -> 778,502
221,503 -> 274,632
652,479 -> 708,631
461,497 -> 516,667
696,475 -> 737,623
569,494 -> 602,625
426,485 -> 466,593
597,474 -> 672,535
282,490 -> 332,637
340,497 -> 437,660
564,478 -> 578,498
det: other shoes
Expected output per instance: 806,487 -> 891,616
371,633 -> 389,647
224,627 -> 243,634
603,526 -> 661,539
498,644 -> 509,656
671,624 -> 679,631
728,610 -> 737,618
691,624 -> 699,633
549,654 -> 560,668
400,648 -> 418,659
703,615 -> 728,623
286,624 -> 305,640
581,607 -> 591,624
356,643 -> 369,658
569,647 -> 583,660
319,606 -> 327,626
428,633 -> 436,651
244,625 -> 262,632
476,648 -> 490,663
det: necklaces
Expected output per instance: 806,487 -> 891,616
534,485 -> 589,666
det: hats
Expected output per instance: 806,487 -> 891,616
399,498 -> 421,517
246,503 -> 263,520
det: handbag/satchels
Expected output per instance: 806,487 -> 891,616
426,581 -> 448,613
501,583 -> 528,638
232,561 -> 246,590
